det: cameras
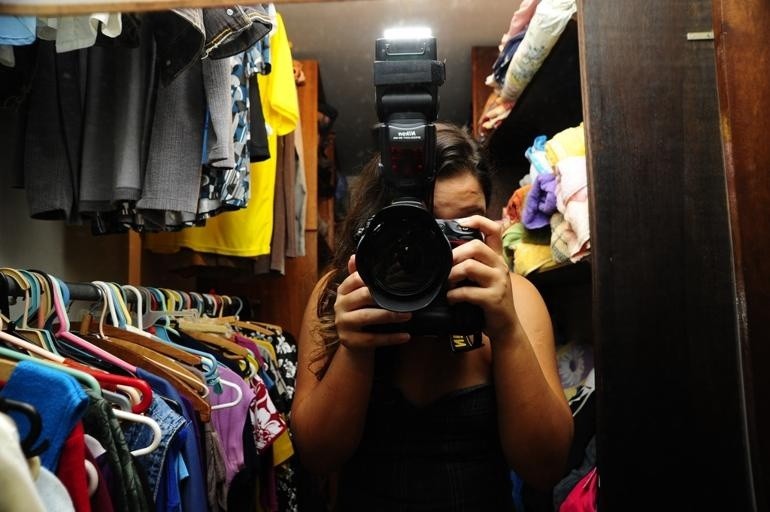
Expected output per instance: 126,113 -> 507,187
353,27 -> 481,351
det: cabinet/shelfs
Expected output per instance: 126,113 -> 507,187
257,54 -> 340,338
470,1 -> 754,512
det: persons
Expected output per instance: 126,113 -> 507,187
289,121 -> 576,511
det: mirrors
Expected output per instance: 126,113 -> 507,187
1,0 -> 769,511
